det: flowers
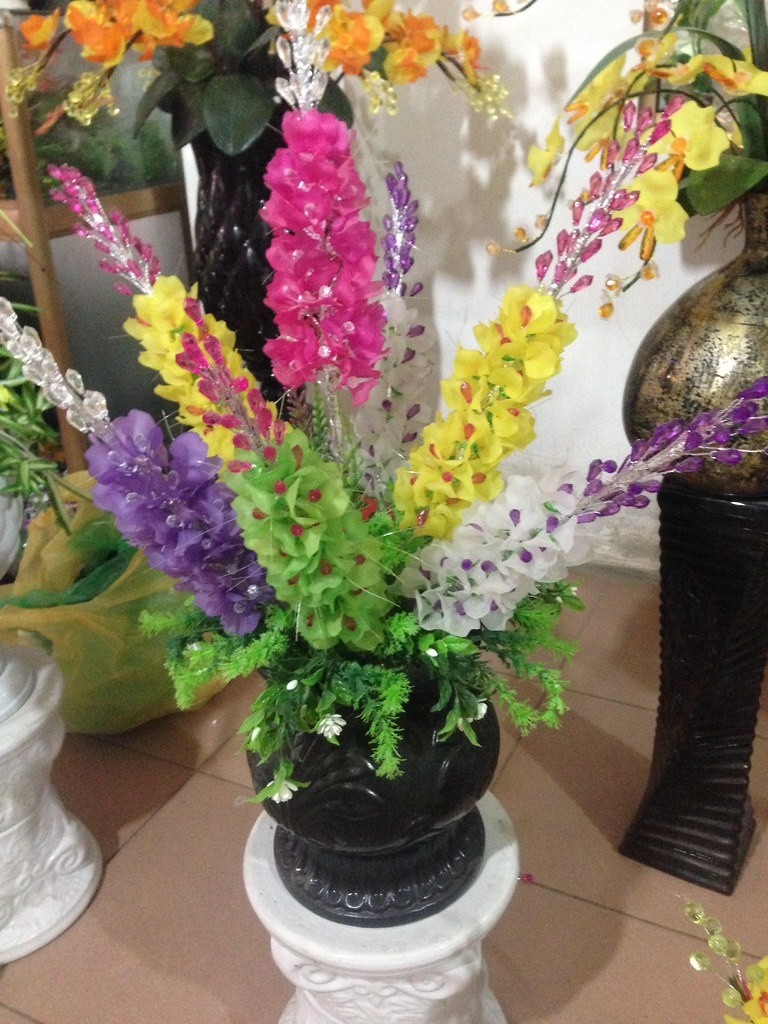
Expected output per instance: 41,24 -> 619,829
0,0 -> 768,806
4,0 -> 537,183
680,896 -> 768,1024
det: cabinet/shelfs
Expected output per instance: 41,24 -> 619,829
0,2 -> 187,475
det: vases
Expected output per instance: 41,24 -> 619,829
243,702 -> 502,929
185,131 -> 304,424
1,640 -> 101,962
241,788 -> 519,1023
620,190 -> 768,492
614,479 -> 767,900
